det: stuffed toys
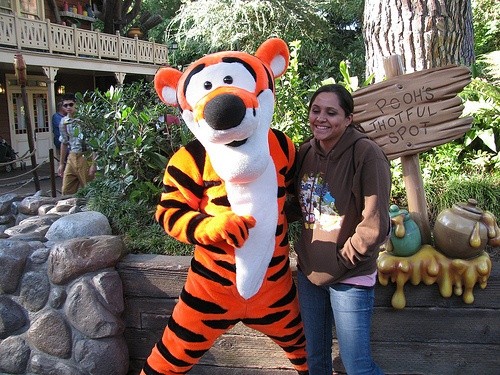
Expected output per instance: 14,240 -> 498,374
137,37 -> 310,375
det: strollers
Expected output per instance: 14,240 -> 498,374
0,135 -> 27,173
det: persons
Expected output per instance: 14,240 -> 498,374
52,101 -> 70,172
284,84 -> 392,375
57,93 -> 97,200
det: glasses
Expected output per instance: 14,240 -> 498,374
62,103 -> 73,108
